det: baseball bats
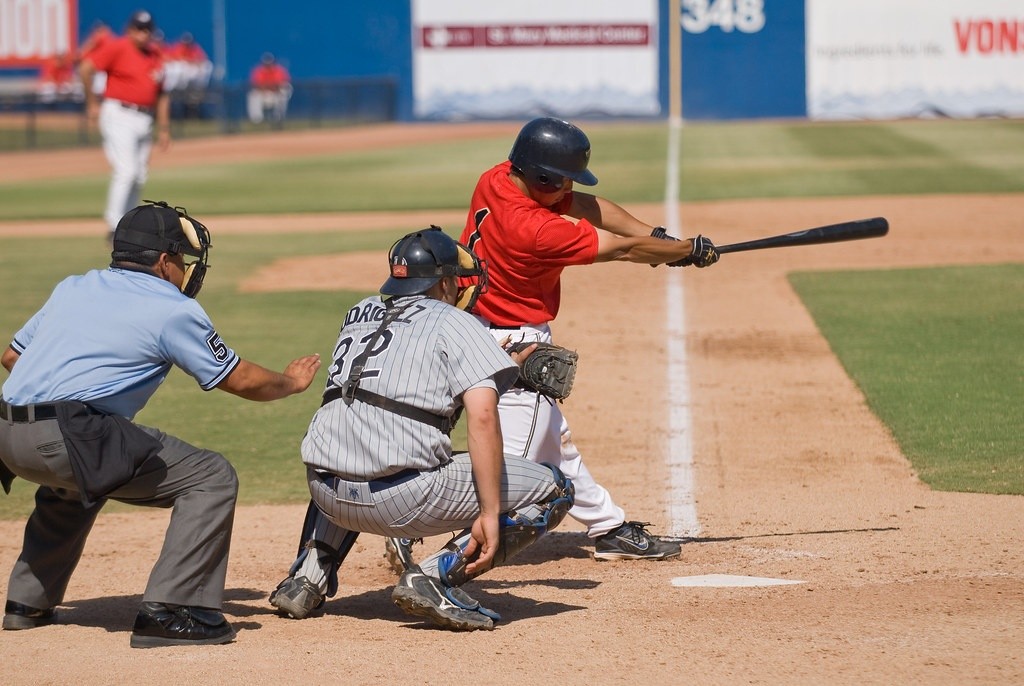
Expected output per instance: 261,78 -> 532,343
649,217 -> 890,268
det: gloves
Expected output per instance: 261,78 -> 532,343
686,234 -> 720,269
650,227 -> 693,267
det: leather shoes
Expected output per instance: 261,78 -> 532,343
131,602 -> 235,648
2,599 -> 57,629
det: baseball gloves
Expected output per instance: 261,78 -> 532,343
506,342 -> 579,400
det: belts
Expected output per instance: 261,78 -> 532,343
0,400 -> 57,421
123,102 -> 153,115
314,468 -> 420,494
490,323 -> 519,330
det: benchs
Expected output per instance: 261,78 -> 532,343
239,77 -> 402,129
0,62 -> 235,151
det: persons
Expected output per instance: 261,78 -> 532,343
70,18 -> 211,132
382,116 -> 721,575
80,10 -> 170,239
247,55 -> 293,123
0,201 -> 323,649
269,226 -> 579,632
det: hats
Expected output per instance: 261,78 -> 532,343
113,205 -> 190,253
130,11 -> 152,30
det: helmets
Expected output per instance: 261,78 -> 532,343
380,230 -> 458,295
508,117 -> 599,194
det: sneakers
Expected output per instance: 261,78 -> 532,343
593,520 -> 681,562
271,576 -> 323,618
384,536 -> 422,573
392,568 -> 494,630
